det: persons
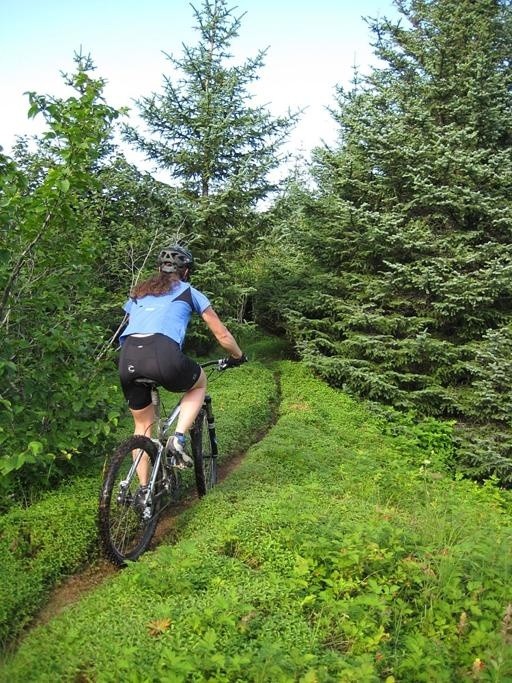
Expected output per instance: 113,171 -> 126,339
116,243 -> 246,521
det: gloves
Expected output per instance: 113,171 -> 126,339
228,352 -> 244,366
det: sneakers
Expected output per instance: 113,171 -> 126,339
165,434 -> 194,468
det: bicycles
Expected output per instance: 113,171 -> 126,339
97,355 -> 250,568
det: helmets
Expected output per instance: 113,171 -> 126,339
157,245 -> 194,273
